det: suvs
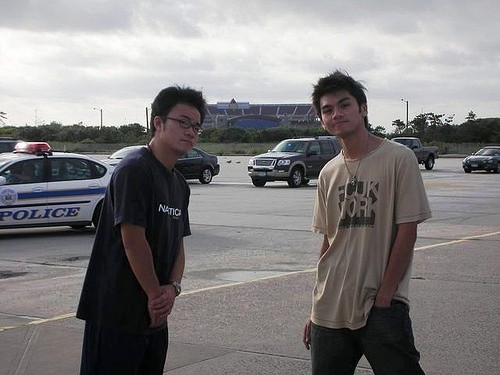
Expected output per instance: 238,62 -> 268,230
248,134 -> 342,188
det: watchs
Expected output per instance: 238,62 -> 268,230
167,279 -> 182,298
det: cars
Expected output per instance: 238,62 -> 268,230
462,146 -> 500,174
95,144 -> 147,170
0,137 -> 116,230
173,146 -> 220,184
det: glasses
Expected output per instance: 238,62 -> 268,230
163,117 -> 205,134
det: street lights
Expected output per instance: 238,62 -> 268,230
401,98 -> 408,128
94,108 -> 102,128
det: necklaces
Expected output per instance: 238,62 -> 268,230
342,132 -> 371,186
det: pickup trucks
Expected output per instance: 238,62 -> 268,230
391,137 -> 440,170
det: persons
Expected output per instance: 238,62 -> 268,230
301,68 -> 433,375
76,86 -> 206,375
